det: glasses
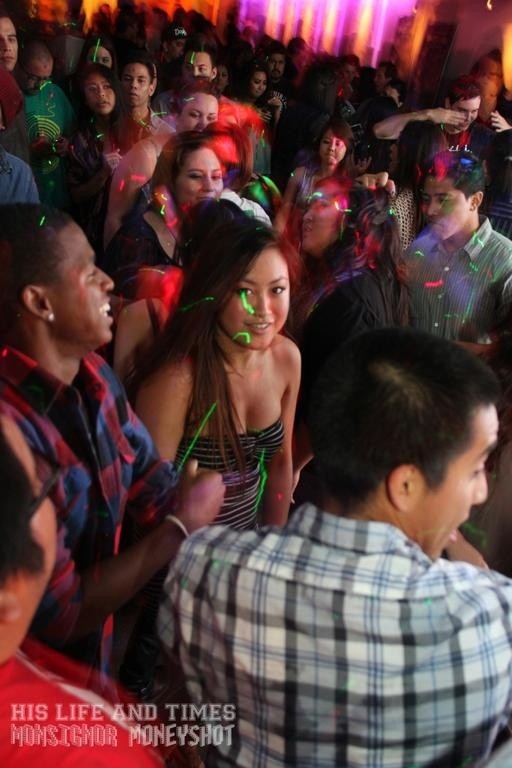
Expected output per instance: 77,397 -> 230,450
26,445 -> 65,523
